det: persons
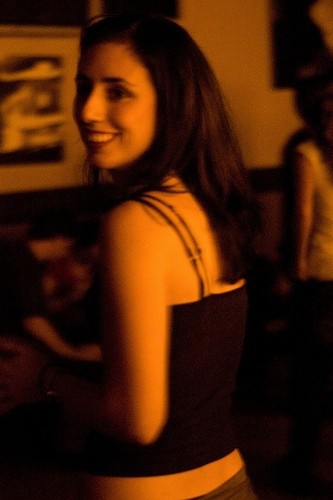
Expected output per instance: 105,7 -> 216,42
0,4 -> 274,500
273,41 -> 333,450
0,188 -> 106,415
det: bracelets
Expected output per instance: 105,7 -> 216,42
36,355 -> 63,402
